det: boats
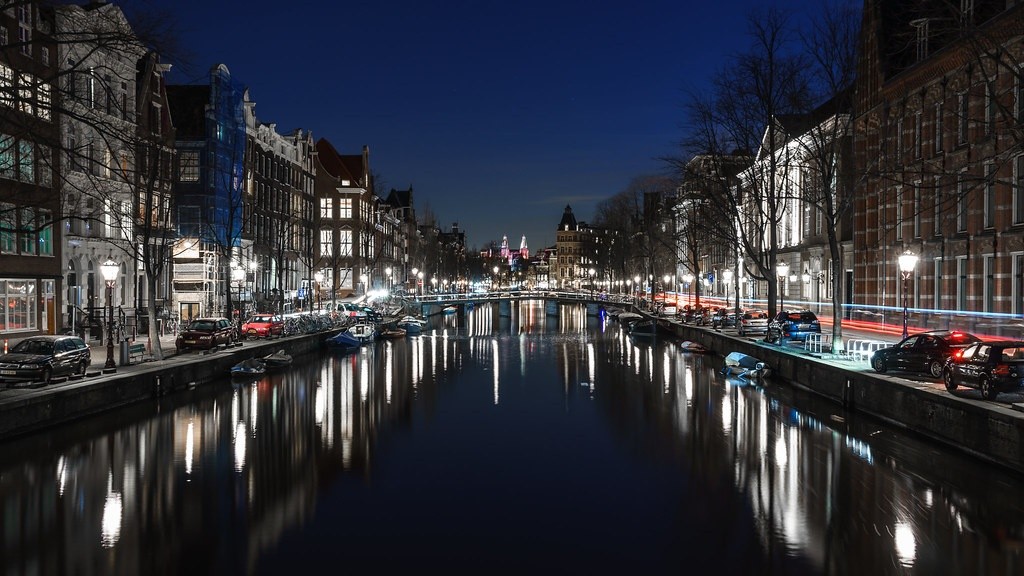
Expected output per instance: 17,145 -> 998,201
230,349 -> 293,381
675,339 -> 709,354
324,330 -> 361,350
381,323 -> 407,337
348,322 -> 376,344
397,314 -> 430,332
443,306 -> 459,313
723,351 -> 773,385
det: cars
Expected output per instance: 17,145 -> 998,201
325,300 -> 384,323
175,317 -> 234,351
241,313 -> 286,340
605,296 -> 822,346
943,340 -> 1024,397
869,329 -> 986,379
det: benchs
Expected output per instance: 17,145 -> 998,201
130,344 -> 146,364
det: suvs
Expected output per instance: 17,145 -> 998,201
0,336 -> 92,386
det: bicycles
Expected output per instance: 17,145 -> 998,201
167,317 -> 180,334
284,311 -> 357,336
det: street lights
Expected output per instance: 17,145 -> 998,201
315,271 -> 324,315
897,249 -> 918,342
723,269 -> 732,308
776,261 -> 789,314
687,273 -> 693,306
411,268 -> 419,302
234,266 -> 247,342
100,256 -> 120,373
